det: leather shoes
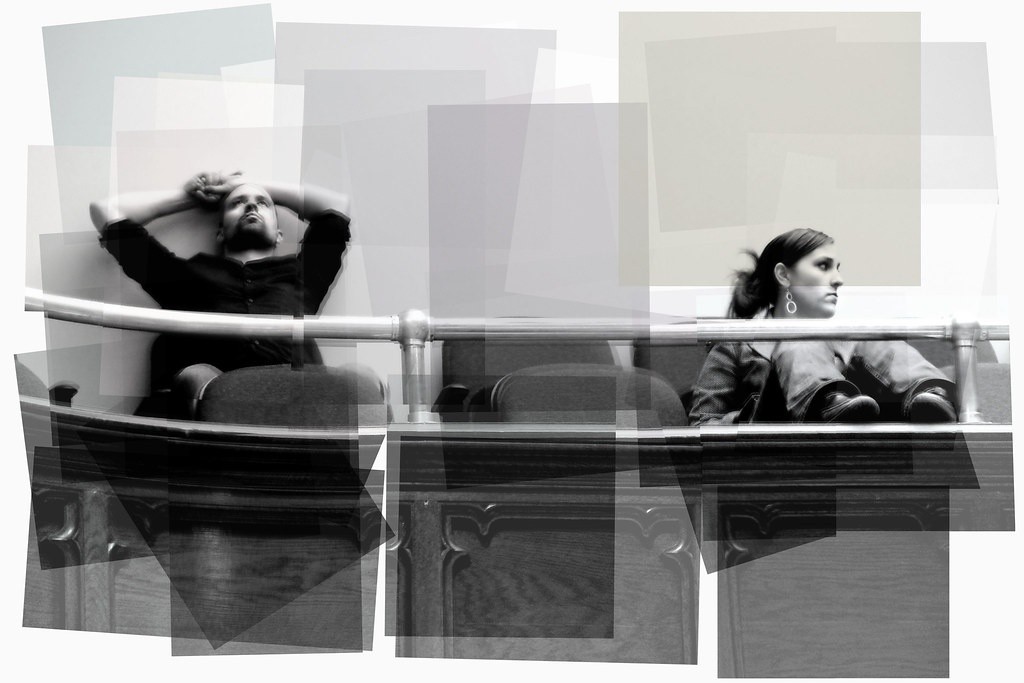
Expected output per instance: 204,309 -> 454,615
805,389 -> 880,423
908,386 -> 956,422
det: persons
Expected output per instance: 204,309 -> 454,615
689,227 -> 957,425
90,168 -> 389,422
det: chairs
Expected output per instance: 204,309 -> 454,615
150,316 -> 1012,425
